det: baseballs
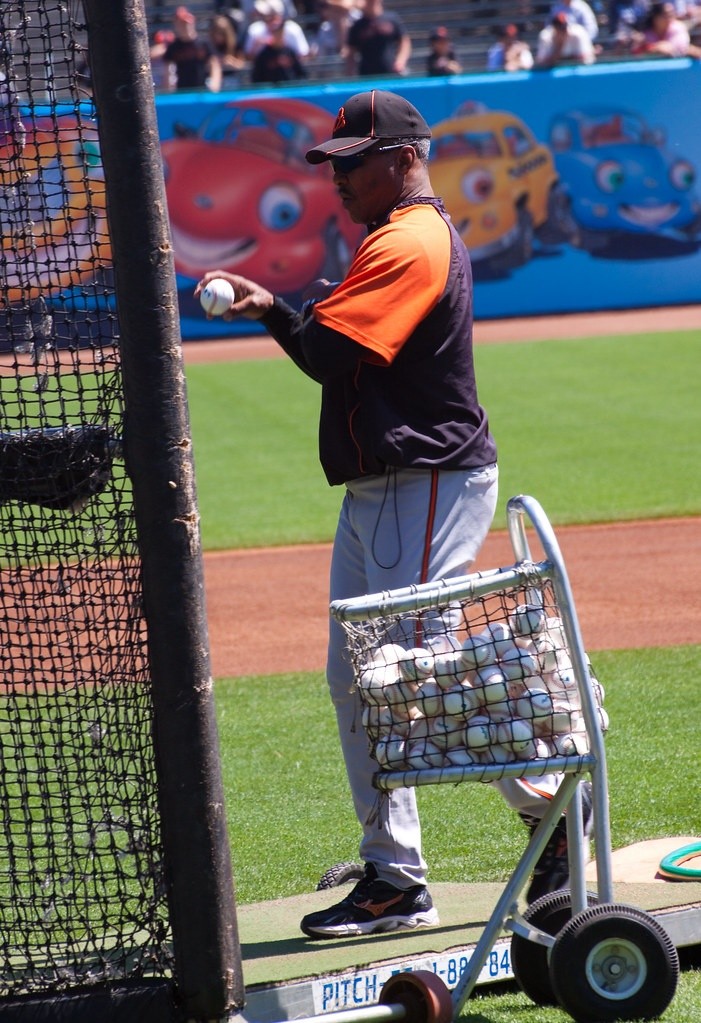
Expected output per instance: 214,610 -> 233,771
358,604 -> 610,771
200,278 -> 237,315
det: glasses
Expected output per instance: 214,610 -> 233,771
331,140 -> 419,174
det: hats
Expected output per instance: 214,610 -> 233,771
304,89 -> 436,164
553,12 -> 574,28
172,8 -> 193,24
501,24 -> 519,41
430,27 -> 451,39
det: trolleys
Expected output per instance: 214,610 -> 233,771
209,488 -> 701,1023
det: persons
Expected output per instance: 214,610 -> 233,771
343,0 -> 411,74
487,24 -> 534,72
161,6 -> 223,93
548,0 -> 701,57
535,13 -> 595,67
211,0 -> 366,81
426,27 -> 462,76
193,90 -> 596,936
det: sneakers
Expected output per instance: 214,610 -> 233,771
301,862 -> 451,939
518,780 -> 596,905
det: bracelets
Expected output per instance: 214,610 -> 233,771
301,298 -> 323,320
